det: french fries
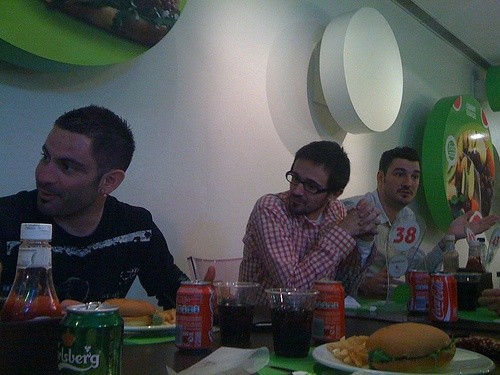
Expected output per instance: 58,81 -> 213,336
328,335 -> 369,368
159,308 -> 177,324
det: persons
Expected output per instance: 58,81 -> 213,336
0,103 -> 231,312
339,147 -> 498,300
236,142 -> 381,307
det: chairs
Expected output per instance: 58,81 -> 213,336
186,255 -> 243,286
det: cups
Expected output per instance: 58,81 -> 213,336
213,281 -> 261,349
455,273 -> 482,311
264,287 -> 320,358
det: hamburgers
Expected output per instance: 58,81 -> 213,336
365,322 -> 457,372
102,298 -> 156,327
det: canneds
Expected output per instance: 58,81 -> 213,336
429,273 -> 458,321
407,269 -> 430,312
175,280 -> 215,350
55,302 -> 124,375
309,280 -> 345,343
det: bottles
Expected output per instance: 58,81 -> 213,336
0,222 -> 63,375
466,237 -> 488,273
442,234 -> 459,274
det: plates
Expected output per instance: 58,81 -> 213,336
312,341 -> 495,375
124,324 -> 176,335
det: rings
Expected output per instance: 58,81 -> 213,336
369,211 -> 371,214
383,284 -> 385,289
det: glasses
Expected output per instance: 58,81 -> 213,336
285,170 -> 333,195
